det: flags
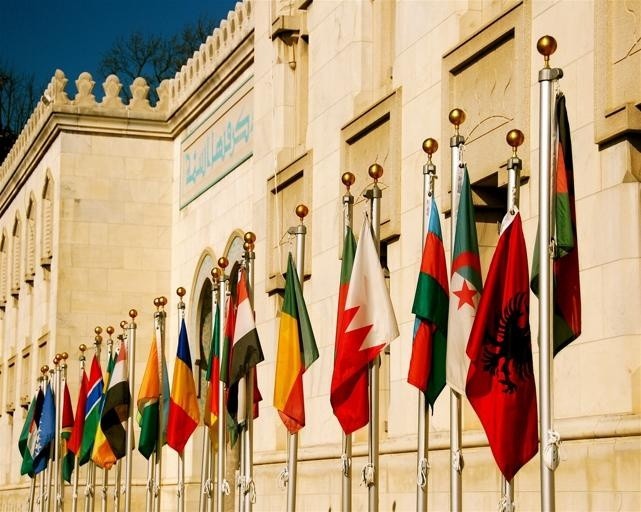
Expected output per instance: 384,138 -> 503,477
445,165 -> 483,398
404,197 -> 449,415
19,336 -> 136,486
202,261 -> 265,456
136,320 -> 200,461
272,252 -> 319,435
330,213 -> 401,437
529,91 -> 581,357
464,205 -> 539,484
336,216 -> 357,338
